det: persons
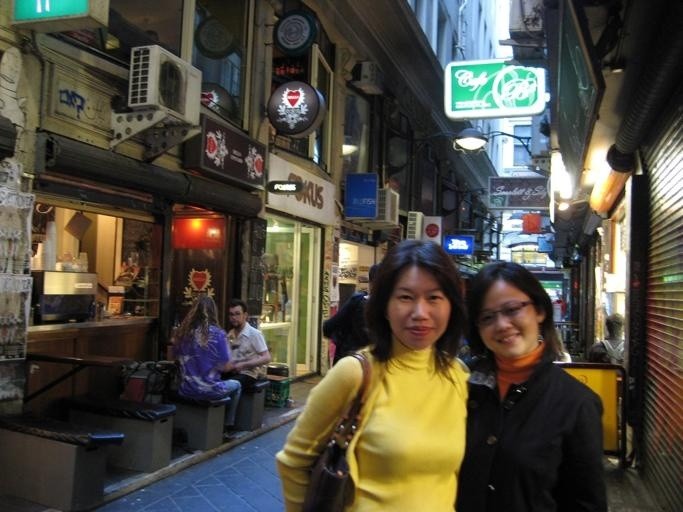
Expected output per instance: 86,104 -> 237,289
218,299 -> 271,391
452,260 -> 607,512
588,315 -> 626,368
554,297 -> 567,318
275,239 -> 472,512
549,331 -> 571,364
171,296 -> 244,441
323,263 -> 382,365
456,338 -> 475,364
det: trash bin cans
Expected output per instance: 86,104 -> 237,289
266,363 -> 289,408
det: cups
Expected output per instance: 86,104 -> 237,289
33,220 -> 57,272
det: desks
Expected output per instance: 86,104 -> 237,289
24,351 -> 134,405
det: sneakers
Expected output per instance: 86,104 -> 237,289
224,430 -> 248,440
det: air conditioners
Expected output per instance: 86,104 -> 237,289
376,188 -> 401,226
405,211 -> 425,244
510,0 -> 545,58
128,44 -> 204,127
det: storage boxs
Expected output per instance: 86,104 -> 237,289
264,376 -> 293,410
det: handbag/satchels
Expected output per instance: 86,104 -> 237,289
305,353 -> 369,512
120,361 -> 176,404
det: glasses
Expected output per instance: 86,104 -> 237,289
477,301 -> 533,326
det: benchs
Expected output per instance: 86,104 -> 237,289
0,380 -> 271,512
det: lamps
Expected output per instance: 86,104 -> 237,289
452,127 -> 533,159
341,132 -> 360,157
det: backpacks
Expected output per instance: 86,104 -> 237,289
594,340 -> 624,364
